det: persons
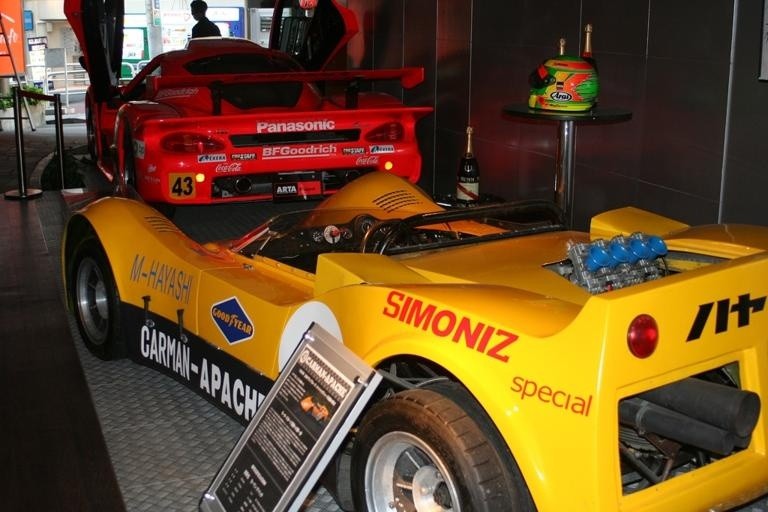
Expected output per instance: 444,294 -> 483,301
188,1 -> 218,39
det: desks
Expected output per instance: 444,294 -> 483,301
499,102 -> 636,226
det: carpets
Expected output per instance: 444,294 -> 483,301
30,185 -> 344,512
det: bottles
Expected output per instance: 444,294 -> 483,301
557,23 -> 599,115
452,124 -> 480,207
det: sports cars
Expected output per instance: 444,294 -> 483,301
60,0 -> 436,222
55,169 -> 768,511
